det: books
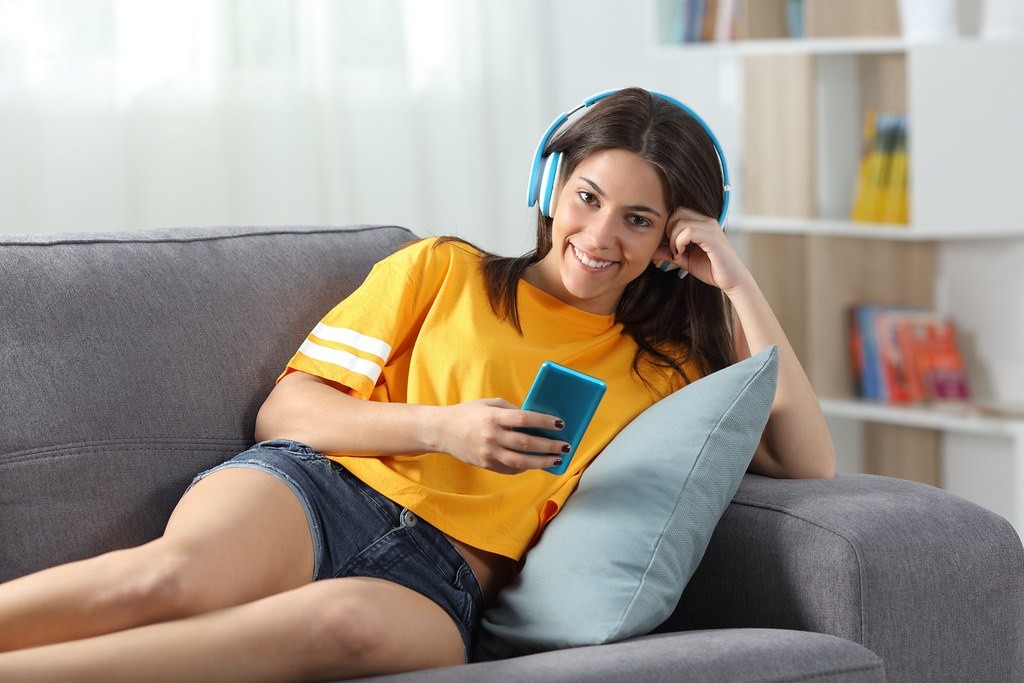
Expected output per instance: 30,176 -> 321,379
676,0 -> 977,415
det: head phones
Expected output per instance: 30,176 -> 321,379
527,89 -> 730,280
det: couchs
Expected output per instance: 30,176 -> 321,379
0,224 -> 1024,683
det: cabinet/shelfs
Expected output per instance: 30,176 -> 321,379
644,0 -> 1024,530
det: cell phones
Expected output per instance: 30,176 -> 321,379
510,363 -> 607,476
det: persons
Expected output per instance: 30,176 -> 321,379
0,88 -> 836,683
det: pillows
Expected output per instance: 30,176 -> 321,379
471,344 -> 779,664
